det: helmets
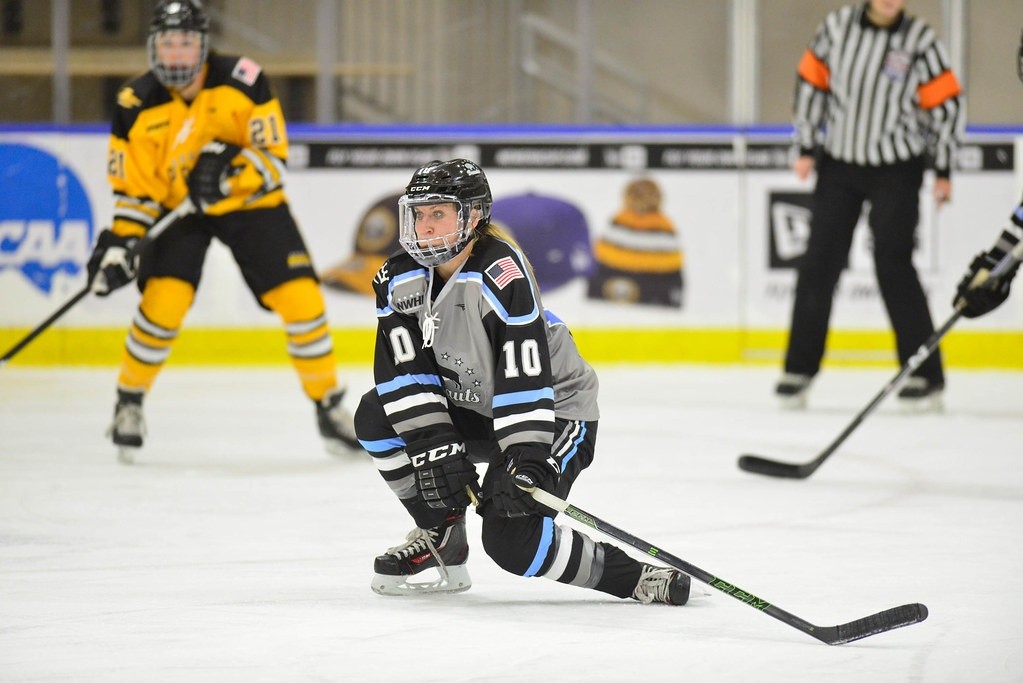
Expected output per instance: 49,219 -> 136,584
147,0 -> 208,88
397,158 -> 491,268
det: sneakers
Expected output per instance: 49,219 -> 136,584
631,565 -> 693,608
776,373 -> 811,406
316,388 -> 371,460
104,386 -> 147,461
899,379 -> 943,411
371,521 -> 470,594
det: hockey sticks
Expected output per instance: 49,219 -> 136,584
1,195 -> 200,363
738,241 -> 1023,479
527,479 -> 928,643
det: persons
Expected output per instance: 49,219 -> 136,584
88,0 -> 373,464
772,0 -> 962,411
953,35 -> 1023,319
354,160 -> 690,606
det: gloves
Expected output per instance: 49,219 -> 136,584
85,229 -> 140,297
185,138 -> 247,212
485,452 -> 556,516
408,430 -> 479,515
953,249 -> 1011,317
